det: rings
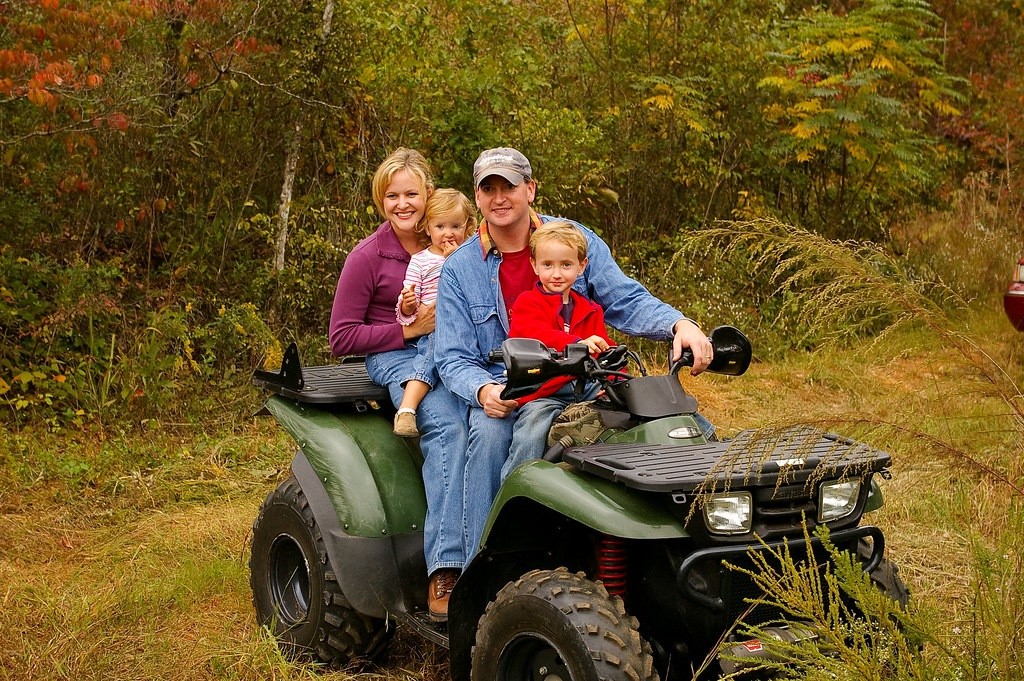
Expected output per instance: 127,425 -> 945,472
707,356 -> 712,359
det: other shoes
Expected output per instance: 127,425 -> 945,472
392,408 -> 419,437
427,569 -> 460,623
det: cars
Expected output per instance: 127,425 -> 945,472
1004,256 -> 1024,332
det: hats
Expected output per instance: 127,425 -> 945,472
472,147 -> 532,191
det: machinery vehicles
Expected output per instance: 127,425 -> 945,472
249,327 -> 924,680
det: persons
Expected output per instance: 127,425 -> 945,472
500,220 -> 632,490
435,146 -> 714,577
329,146 -> 466,620
393,187 -> 480,437
